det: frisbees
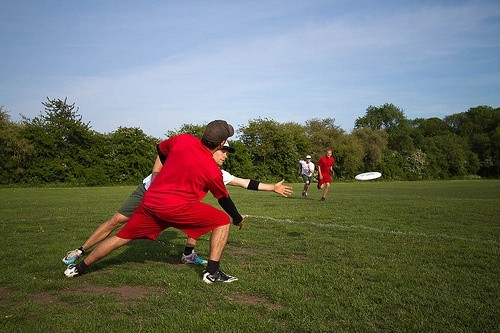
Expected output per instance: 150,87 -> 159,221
354,172 -> 382,180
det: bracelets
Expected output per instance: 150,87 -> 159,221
247,179 -> 260,191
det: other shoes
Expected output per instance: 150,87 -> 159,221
302,191 -> 307,196
321,197 -> 325,200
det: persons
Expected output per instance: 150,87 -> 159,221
298,155 -> 314,196
317,149 -> 334,200
64,121 -> 248,283
61,140 -> 293,265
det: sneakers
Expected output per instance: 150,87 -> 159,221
64,264 -> 78,278
203,269 -> 237,283
181,250 -> 208,264
62,249 -> 82,265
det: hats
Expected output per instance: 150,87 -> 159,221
219,139 -> 235,153
306,155 -> 311,159
205,120 -> 234,143
